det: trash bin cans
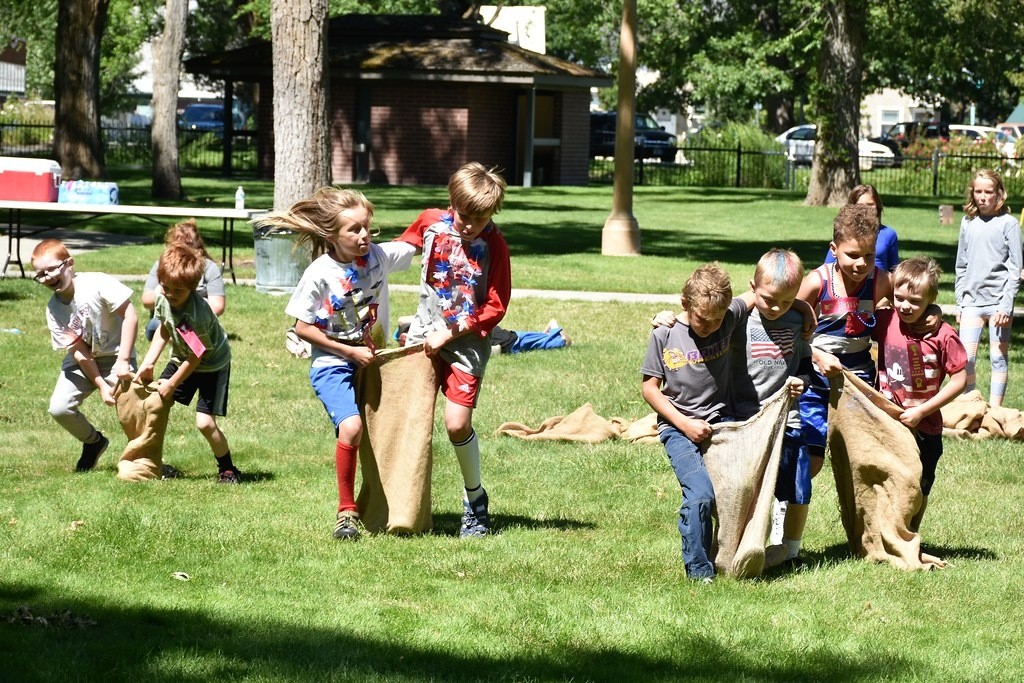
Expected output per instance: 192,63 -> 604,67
253,221 -> 312,295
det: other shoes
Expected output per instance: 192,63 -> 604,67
770,498 -> 788,546
218,466 -> 240,484
784,557 -> 803,569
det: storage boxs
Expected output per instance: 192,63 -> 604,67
0,157 -> 61,201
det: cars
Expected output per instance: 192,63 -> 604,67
997,123 -> 1024,139
177,104 -> 227,132
100,111 -> 152,143
233,111 -> 246,131
0,100 -> 56,132
923,125 -> 1022,168
774,124 -> 895,172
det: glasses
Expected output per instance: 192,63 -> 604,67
33,259 -> 67,284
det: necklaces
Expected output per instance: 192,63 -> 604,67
829,260 -> 876,329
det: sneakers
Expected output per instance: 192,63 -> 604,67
76,431 -> 109,472
333,511 -> 359,539
459,487 -> 489,540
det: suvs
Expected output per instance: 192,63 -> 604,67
869,121 -> 949,155
589,112 -> 679,162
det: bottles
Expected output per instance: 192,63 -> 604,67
57,179 -> 119,205
235,186 -> 245,211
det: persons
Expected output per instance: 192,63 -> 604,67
870,257 -> 968,570
954,169 -> 1022,406
391,160 -> 512,541
142,218 -> 225,355
131,244 -> 240,484
397,315 -> 569,353
639,263 -> 819,583
246,186 -> 422,540
823,184 -> 899,279
766,203 -> 942,561
652,246 -> 814,578
31,239 -> 182,479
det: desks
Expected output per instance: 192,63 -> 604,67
0,201 -> 267,284
